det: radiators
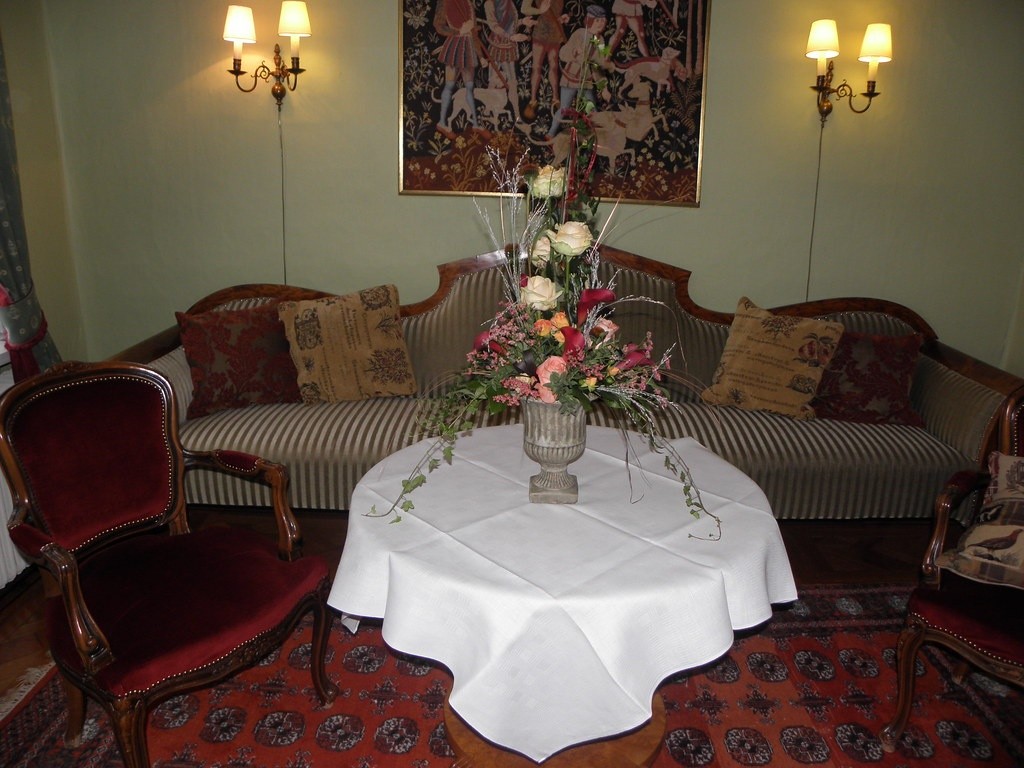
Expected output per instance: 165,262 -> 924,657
0,467 -> 32,593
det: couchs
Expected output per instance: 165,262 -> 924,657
98,241 -> 1024,527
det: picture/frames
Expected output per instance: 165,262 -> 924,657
397,0 -> 712,207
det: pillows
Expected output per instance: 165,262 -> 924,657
934,451 -> 1024,592
702,295 -> 845,420
277,284 -> 418,406
811,330 -> 927,425
174,310 -> 306,422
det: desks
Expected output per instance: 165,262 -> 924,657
327,424 -> 799,767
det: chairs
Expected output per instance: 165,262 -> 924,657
881,393 -> 1023,743
0,361 -> 333,768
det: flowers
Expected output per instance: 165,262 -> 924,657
361,19 -> 722,543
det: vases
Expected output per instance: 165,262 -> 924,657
520,396 -> 588,504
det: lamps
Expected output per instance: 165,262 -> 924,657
805,18 -> 893,119
223,1 -> 312,108
